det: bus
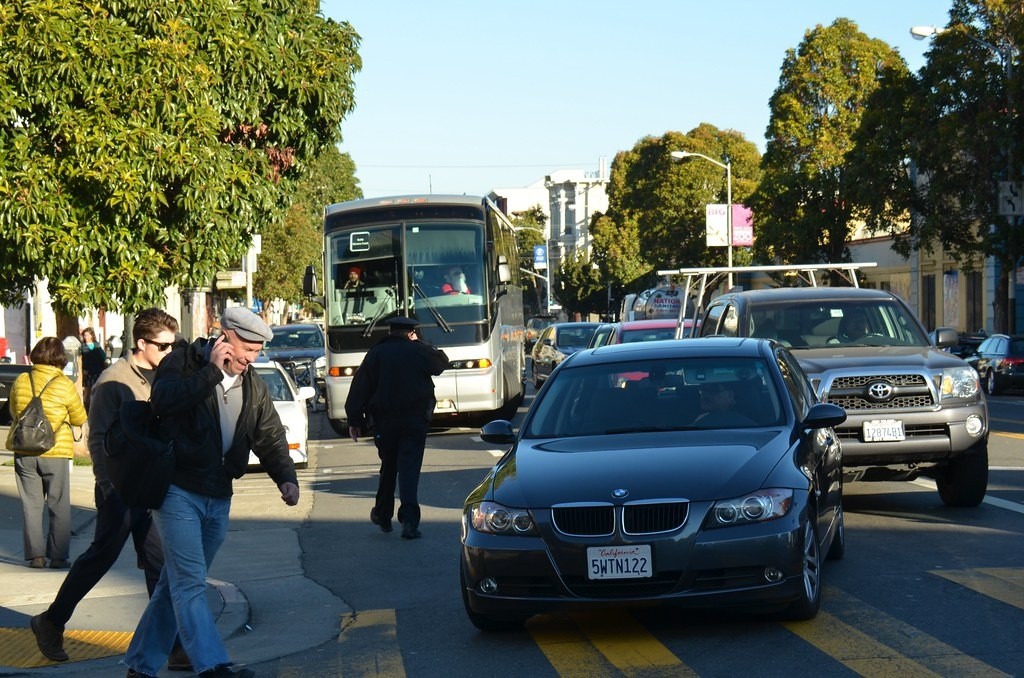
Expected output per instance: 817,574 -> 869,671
303,192 -> 528,437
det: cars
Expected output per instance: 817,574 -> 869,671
529,321 -> 608,390
961,331 -> 1024,397
581,318 -> 704,390
261,321 -> 328,412
248,356 -> 317,473
456,334 -> 849,634
0,363 -> 37,427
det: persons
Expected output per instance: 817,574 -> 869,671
79,327 -> 106,414
343,267 -> 364,289
441,266 -> 471,295
696,382 -> 734,421
120,306 -> 299,678
303,334 -> 319,345
29,308 -> 193,670
9,337 -> 87,569
827,311 -> 867,344
344,317 -> 449,538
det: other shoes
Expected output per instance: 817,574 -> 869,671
31,612 -> 69,662
167,651 -> 195,670
29,557 -> 47,568
200,663 -> 254,678
127,670 -> 156,678
49,559 -> 71,568
370,507 -> 393,532
400,521 -> 422,539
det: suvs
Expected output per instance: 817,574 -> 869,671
693,283 -> 992,509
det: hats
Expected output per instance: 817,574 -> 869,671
221,307 -> 274,342
349,267 -> 361,278
384,316 -> 418,331
845,306 -> 866,318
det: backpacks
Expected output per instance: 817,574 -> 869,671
5,372 -> 68,454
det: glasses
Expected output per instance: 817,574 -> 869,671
699,389 -> 726,397
142,338 -> 177,351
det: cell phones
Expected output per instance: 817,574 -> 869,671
217,334 -> 230,365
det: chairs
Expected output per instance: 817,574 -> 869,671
558,333 -> 573,345
582,388 -> 635,431
805,317 -> 845,345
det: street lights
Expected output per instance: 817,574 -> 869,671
514,227 -> 551,316
671,150 -> 735,294
